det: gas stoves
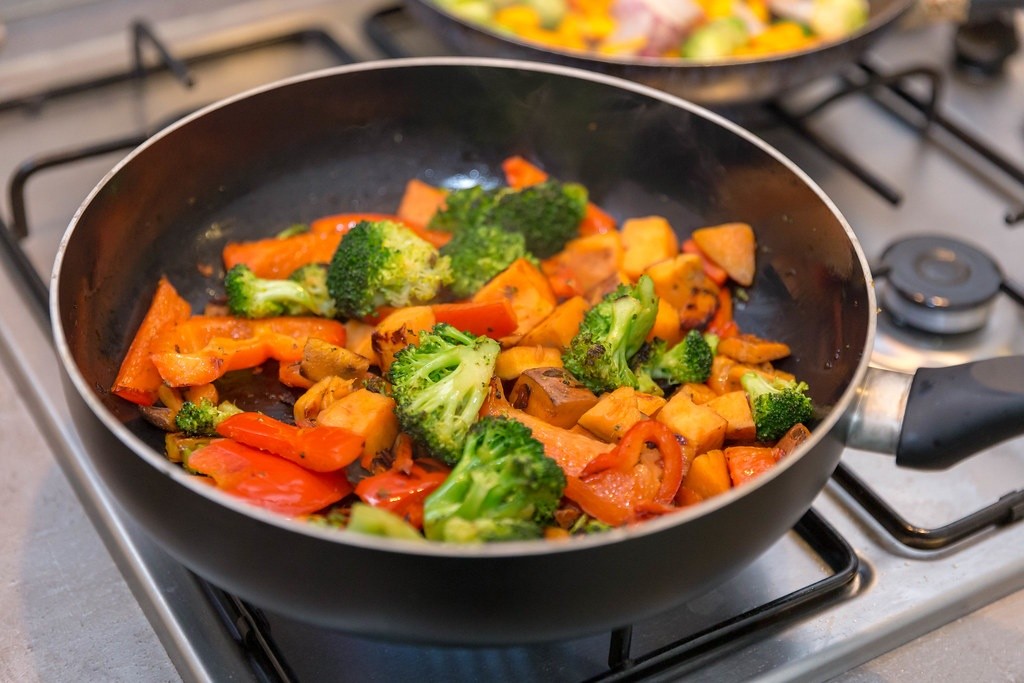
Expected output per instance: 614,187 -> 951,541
0,0 -> 1024,683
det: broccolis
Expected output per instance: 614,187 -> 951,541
176,180 -> 812,545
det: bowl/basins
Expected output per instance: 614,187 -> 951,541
48,56 -> 879,647
408,0 -> 914,107
